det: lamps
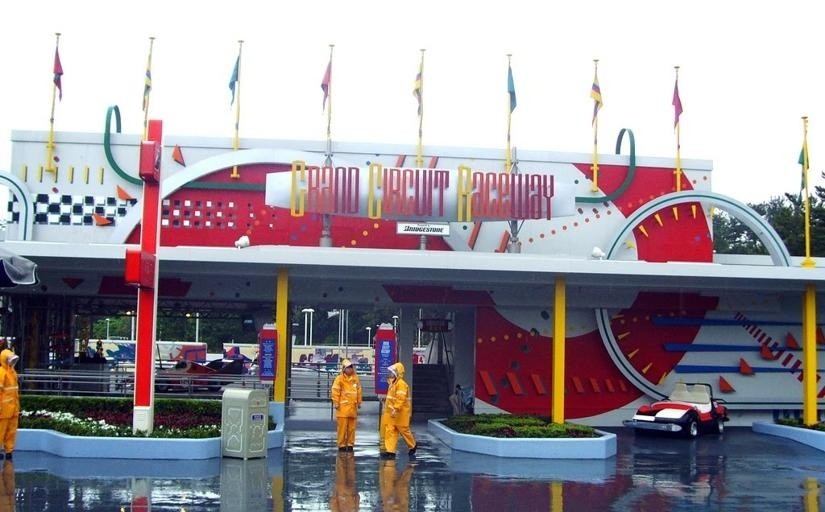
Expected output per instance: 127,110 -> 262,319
234,234 -> 251,250
591,246 -> 607,260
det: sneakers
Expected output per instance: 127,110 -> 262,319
379,443 -> 418,459
338,445 -> 353,452
0,453 -> 13,460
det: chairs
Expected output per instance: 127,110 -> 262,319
670,379 -> 711,406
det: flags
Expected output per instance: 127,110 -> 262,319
53,44 -> 64,100
142,48 -> 152,114
590,69 -> 604,128
413,62 -> 424,115
797,142 -> 810,193
228,55 -> 239,107
507,66 -> 517,115
319,59 -> 331,114
671,80 -> 684,134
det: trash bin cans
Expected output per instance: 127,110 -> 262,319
221,386 -> 270,461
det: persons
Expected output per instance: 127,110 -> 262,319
0,348 -> 28,459
378,458 -> 414,511
0,459 -> 17,512
329,449 -> 360,511
379,361 -> 417,459
330,358 -> 362,451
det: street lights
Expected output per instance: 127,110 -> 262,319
392,316 -> 398,333
302,309 -> 315,346
366,326 -> 371,347
